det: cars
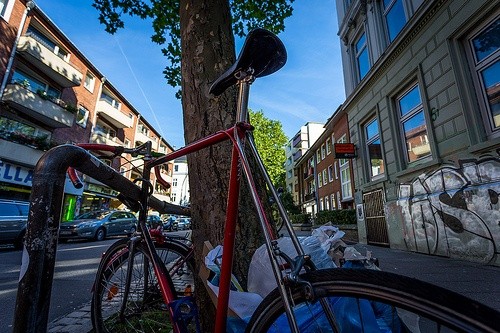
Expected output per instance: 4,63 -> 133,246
176,218 -> 190,231
0,199 -> 31,247
58,210 -> 138,241
136,215 -> 164,233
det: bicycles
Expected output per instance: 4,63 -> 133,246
67,27 -> 500,333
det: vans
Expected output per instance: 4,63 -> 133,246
161,216 -> 179,232
